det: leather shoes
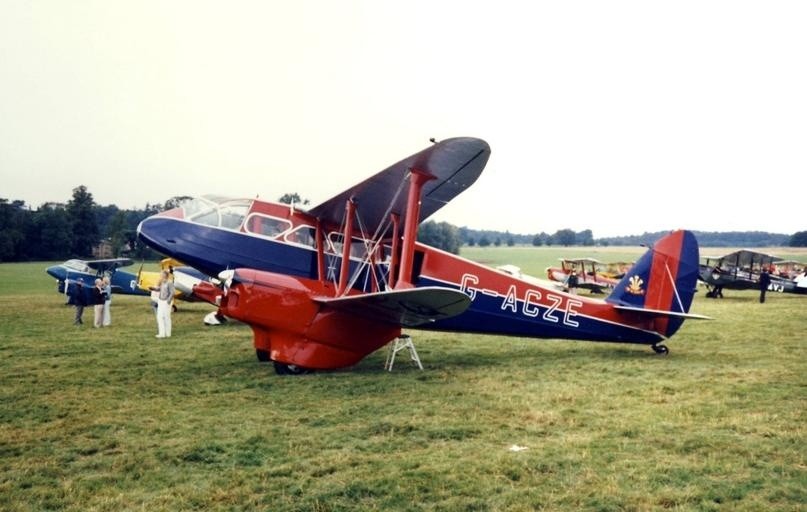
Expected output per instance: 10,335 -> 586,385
155,333 -> 171,338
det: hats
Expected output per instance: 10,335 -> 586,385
77,278 -> 84,281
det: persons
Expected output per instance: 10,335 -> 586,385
100,276 -> 111,327
73,277 -> 84,324
709,279 -> 725,298
149,271 -> 175,338
561,268 -> 578,297
759,267 -> 771,303
94,279 -> 108,328
167,265 -> 178,312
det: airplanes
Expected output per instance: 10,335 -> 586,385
696,249 -> 807,298
136,137 -> 716,375
46,257 -> 155,306
545,258 -> 638,294
